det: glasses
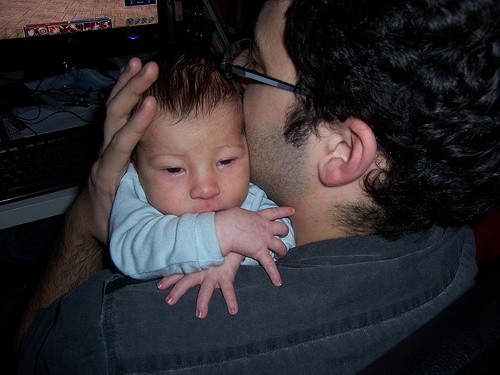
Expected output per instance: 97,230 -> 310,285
219,34 -> 314,100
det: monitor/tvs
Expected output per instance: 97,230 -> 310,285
1,25 -> 160,144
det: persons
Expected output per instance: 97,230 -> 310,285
1,0 -> 499,375
107,43 -> 296,317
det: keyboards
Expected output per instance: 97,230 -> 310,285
0,123 -> 104,211
